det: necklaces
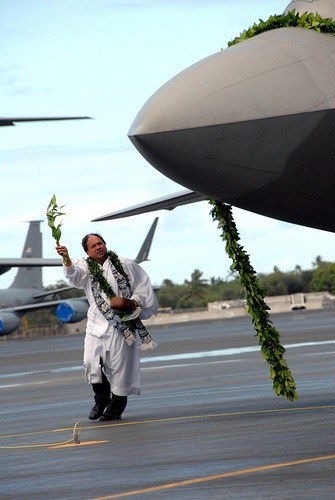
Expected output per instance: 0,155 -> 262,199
86,250 -> 132,300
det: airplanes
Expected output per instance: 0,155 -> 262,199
0,258 -> 65,276
0,116 -> 93,127
91,0 -> 335,233
0,216 -> 158,337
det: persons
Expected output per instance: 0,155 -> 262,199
53,233 -> 155,423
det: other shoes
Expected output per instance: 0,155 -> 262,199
88,399 -> 111,420
99,410 -> 119,421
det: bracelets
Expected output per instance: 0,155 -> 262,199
125,300 -> 136,314
